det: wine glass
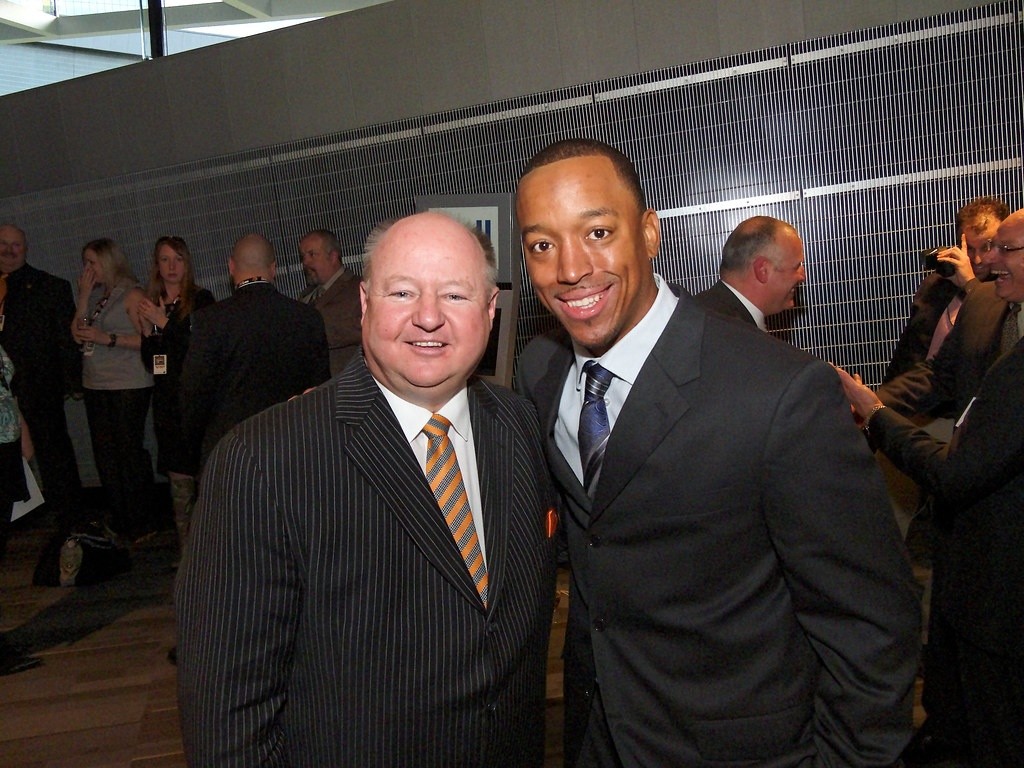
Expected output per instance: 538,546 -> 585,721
77,318 -> 94,352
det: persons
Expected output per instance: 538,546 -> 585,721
177,212 -> 560,768
515,137 -> 920,768
0,223 -> 365,580
692,197 -> 1024,768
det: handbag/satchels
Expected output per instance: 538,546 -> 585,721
32,519 -> 134,587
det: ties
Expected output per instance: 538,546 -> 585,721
1001,303 -> 1021,354
578,363 -> 613,503
309,287 -> 324,306
421,415 -> 488,610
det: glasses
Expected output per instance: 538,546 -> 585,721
985,239 -> 1024,255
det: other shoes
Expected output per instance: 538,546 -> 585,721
0,644 -> 23,672
125,520 -> 149,536
108,516 -> 133,532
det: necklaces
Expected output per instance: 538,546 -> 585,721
235,276 -> 269,291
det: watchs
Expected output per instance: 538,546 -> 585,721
107,334 -> 117,347
861,406 -> 886,436
